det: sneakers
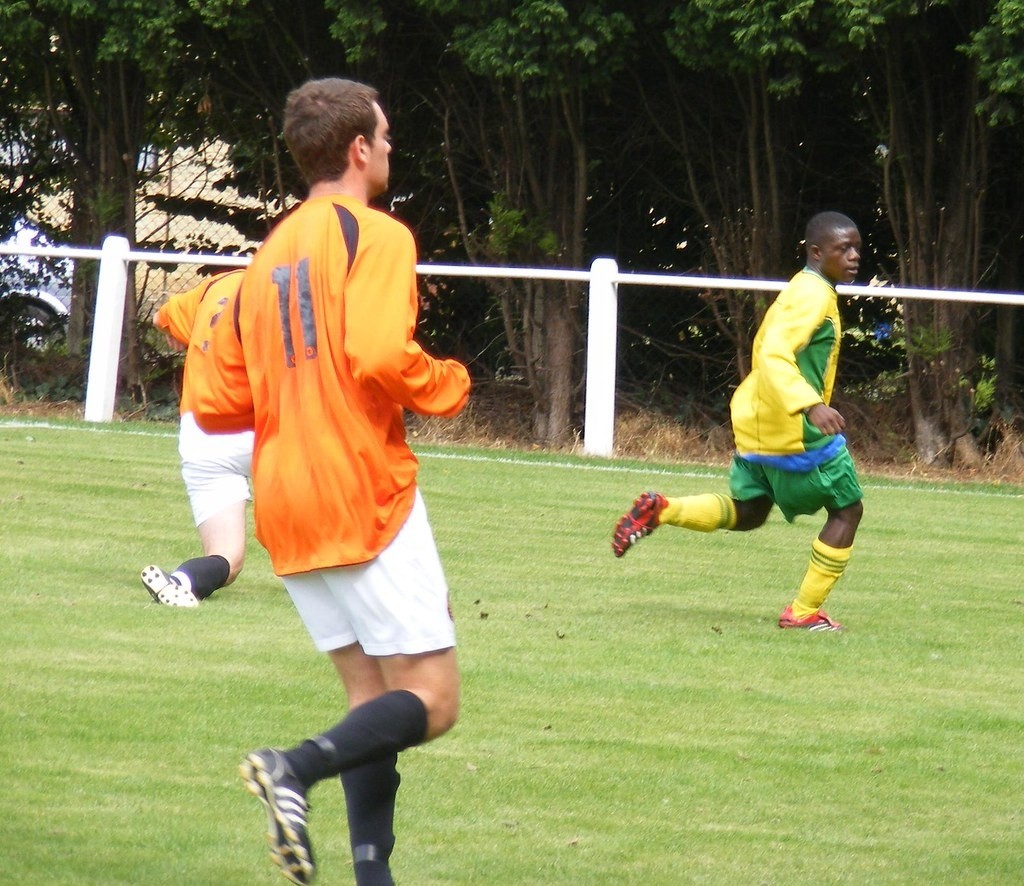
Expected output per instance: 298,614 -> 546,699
779,604 -> 840,631
141,565 -> 199,608
612,490 -> 669,558
240,747 -> 315,886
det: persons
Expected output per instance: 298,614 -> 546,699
613,212 -> 864,631
140,269 -> 253,606
192,77 -> 470,886
